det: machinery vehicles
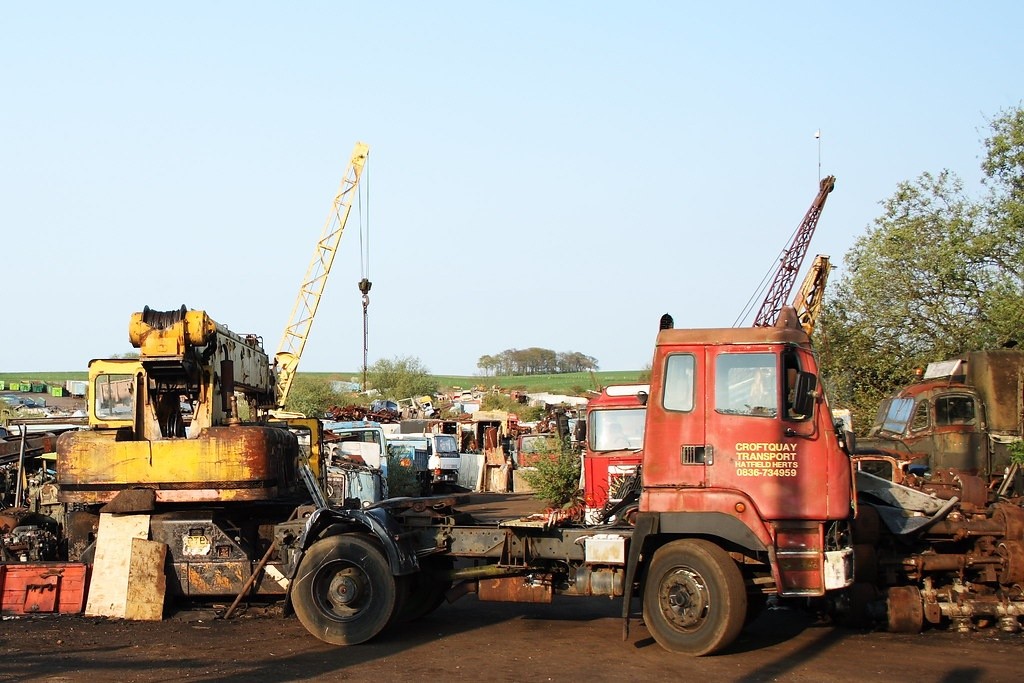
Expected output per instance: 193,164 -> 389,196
1,143 -> 1024,657
